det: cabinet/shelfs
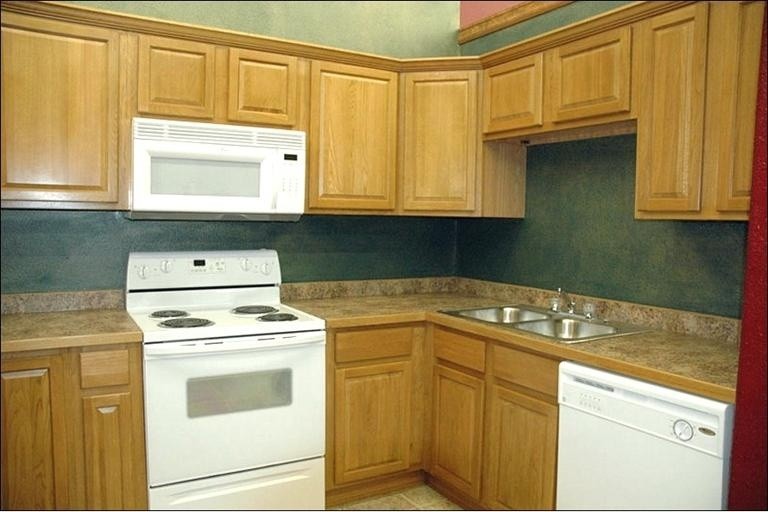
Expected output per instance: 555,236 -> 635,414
0,10 -> 120,203
1,343 -> 148,511
426,321 -> 561,511
632,0 -> 765,222
305,58 -> 527,219
131,34 -> 304,131
324,321 -> 425,511
482,24 -> 633,145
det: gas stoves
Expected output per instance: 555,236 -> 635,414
124,249 -> 325,342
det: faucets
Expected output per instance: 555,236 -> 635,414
557,286 -> 577,313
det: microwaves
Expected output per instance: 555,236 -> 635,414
127,117 -> 306,223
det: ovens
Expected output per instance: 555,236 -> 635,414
141,331 -> 326,510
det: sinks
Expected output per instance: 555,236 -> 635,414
459,307 -> 548,323
516,319 -> 614,339
